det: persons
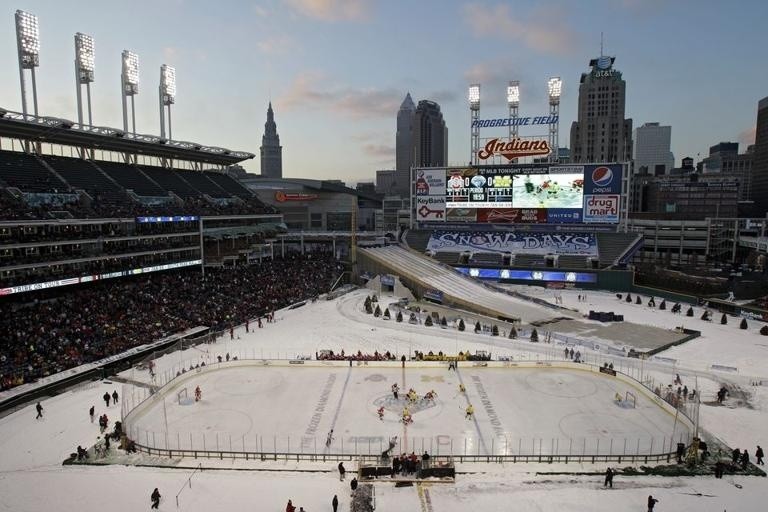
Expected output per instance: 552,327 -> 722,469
151,488 -> 161,508
389,436 -> 397,450
378,407 -> 384,421
464,405 -> 474,420
77,391 -> 137,459
299,507 -> 305,512
677,438 -> 764,478
604,362 -> 613,369
1,186 -> 348,389
668,374 -> 701,403
36,401 -> 43,418
339,462 -> 345,481
355,351 -> 471,371
326,430 -> 334,446
391,383 -> 437,425
605,467 -> 613,487
350,477 -> 357,497
392,451 -> 430,477
718,386 -> 728,402
286,500 -> 295,512
564,347 -> 580,360
332,495 -> 338,512
194,385 -> 201,401
647,496 -> 655,512
459,384 -> 465,392
